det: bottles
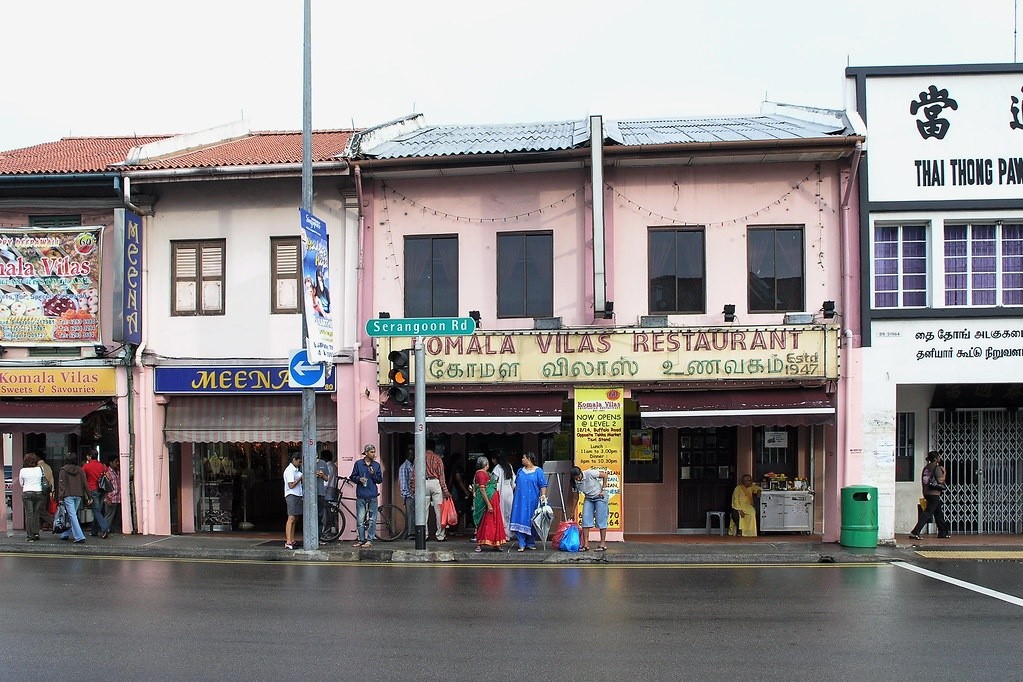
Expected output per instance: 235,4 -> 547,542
362,473 -> 368,486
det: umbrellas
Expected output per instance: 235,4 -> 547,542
531,495 -> 554,550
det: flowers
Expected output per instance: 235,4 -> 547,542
764,471 -> 781,480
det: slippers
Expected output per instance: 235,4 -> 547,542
938,534 -> 951,538
352,542 -> 365,547
578,545 -> 590,552
360,542 -> 373,548
908,535 -> 923,540
594,545 -> 609,552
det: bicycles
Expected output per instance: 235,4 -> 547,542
318,476 -> 408,542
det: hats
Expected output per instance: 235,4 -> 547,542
360,444 -> 376,455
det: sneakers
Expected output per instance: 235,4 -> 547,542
24,534 -> 39,543
285,540 -> 301,550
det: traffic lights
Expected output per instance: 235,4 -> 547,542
388,349 -> 410,406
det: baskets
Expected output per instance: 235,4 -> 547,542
324,486 -> 340,503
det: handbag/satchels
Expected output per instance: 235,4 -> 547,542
39,465 -> 52,495
550,520 -> 585,553
440,496 -> 459,527
917,498 -> 934,523
927,464 -> 947,490
52,504 -> 71,534
97,463 -> 115,493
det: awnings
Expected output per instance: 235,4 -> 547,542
160,394 -> 338,444
375,393 -> 565,436
634,390 -> 834,430
0,397 -> 109,435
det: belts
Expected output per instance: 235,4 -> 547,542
426,477 -> 435,480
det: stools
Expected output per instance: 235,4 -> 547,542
706,511 -> 725,536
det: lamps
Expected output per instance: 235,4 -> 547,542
821,301 -> 835,319
722,304 -> 737,323
604,301 -> 614,320
469,310 -> 481,329
0,345 -> 7,357
95,344 -> 106,355
380,311 -> 390,318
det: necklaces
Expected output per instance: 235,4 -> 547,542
223,461 -> 232,475
211,460 -> 219,473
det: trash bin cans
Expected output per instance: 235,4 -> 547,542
840,485 -> 879,548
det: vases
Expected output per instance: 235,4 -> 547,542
772,480 -> 778,491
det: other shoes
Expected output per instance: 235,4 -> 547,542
737,531 -> 742,537
516,545 -> 537,552
474,545 -> 482,553
73,539 -> 86,544
59,535 -> 68,540
88,532 -> 98,536
319,541 -> 327,546
437,536 -> 448,542
492,546 -> 504,552
101,529 -> 113,539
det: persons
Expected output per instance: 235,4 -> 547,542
730,472 -> 763,537
281,451 -> 304,549
18,453 -> 44,542
907,451 -> 953,539
35,454 -> 54,531
472,447 -> 549,551
305,270 -> 329,318
82,448 -> 112,538
58,451 -> 90,542
349,442 -> 382,547
569,467 -> 609,552
315,449 -> 340,547
398,440 -> 453,542
102,456 -> 122,532
